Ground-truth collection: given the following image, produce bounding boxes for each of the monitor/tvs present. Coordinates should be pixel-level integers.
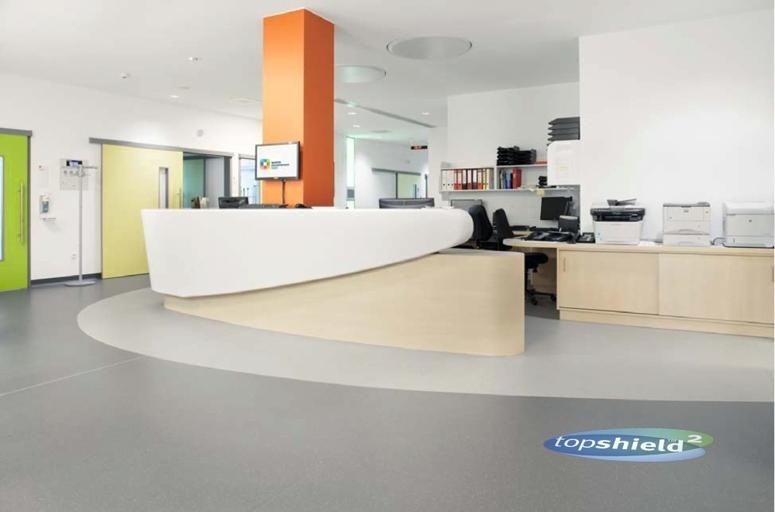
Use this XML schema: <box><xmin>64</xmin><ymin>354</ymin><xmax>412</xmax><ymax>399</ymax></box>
<box><xmin>219</xmin><ymin>197</ymin><xmax>249</xmax><ymax>208</ymax></box>
<box><xmin>379</xmin><ymin>197</ymin><xmax>435</xmax><ymax>209</ymax></box>
<box><xmin>540</xmin><ymin>196</ymin><xmax>570</xmax><ymax>220</ymax></box>
<box><xmin>451</xmin><ymin>199</ymin><xmax>482</xmax><ymax>212</ymax></box>
<box><xmin>255</xmin><ymin>142</ymin><xmax>300</xmax><ymax>180</ymax></box>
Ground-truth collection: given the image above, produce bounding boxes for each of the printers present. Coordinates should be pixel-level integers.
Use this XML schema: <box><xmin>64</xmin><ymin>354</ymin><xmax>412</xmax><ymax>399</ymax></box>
<box><xmin>590</xmin><ymin>207</ymin><xmax>645</xmax><ymax>244</ymax></box>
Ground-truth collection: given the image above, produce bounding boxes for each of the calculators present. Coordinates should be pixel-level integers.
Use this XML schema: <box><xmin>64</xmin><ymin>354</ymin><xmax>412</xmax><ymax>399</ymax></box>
<box><xmin>577</xmin><ymin>233</ymin><xmax>595</xmax><ymax>243</ymax></box>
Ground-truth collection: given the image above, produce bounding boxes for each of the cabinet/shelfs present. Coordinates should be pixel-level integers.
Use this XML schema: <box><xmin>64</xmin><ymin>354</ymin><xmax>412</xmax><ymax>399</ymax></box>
<box><xmin>656</xmin><ymin>254</ymin><xmax>772</xmax><ymax>341</ymax></box>
<box><xmin>439</xmin><ymin>160</ymin><xmax>576</xmax><ymax>192</ymax></box>
<box><xmin>557</xmin><ymin>251</ymin><xmax>657</xmax><ymax>329</ymax></box>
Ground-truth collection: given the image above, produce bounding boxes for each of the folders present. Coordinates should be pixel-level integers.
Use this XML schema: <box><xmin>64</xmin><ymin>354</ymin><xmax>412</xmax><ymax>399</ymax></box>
<box><xmin>441</xmin><ymin>169</ymin><xmax>494</xmax><ymax>190</ymax></box>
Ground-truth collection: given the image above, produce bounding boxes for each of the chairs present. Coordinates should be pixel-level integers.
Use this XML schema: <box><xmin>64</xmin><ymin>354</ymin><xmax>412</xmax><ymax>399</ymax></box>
<box><xmin>467</xmin><ymin>206</ymin><xmax>555</xmax><ymax>305</ymax></box>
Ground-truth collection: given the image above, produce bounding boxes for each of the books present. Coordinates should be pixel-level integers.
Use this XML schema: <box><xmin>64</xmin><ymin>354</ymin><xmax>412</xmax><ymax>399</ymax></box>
<box><xmin>442</xmin><ymin>168</ymin><xmax>522</xmax><ymax>190</ymax></box>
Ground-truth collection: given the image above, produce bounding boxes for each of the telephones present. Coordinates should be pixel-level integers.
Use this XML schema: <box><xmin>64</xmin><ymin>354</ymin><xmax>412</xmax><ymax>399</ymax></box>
<box><xmin>527</xmin><ymin>231</ymin><xmax>546</xmax><ymax>240</ymax></box>
